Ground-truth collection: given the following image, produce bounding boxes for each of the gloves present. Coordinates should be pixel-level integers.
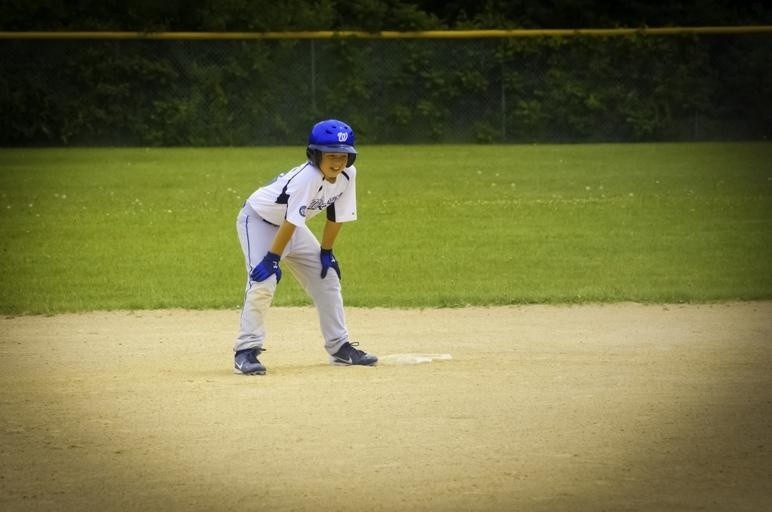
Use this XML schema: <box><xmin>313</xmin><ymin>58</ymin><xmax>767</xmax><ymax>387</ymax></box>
<box><xmin>250</xmin><ymin>252</ymin><xmax>282</xmax><ymax>283</ymax></box>
<box><xmin>319</xmin><ymin>246</ymin><xmax>342</xmax><ymax>280</ymax></box>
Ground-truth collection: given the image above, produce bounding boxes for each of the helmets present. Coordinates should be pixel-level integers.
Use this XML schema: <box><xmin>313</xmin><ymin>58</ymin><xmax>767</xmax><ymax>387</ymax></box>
<box><xmin>306</xmin><ymin>119</ymin><xmax>359</xmax><ymax>155</ymax></box>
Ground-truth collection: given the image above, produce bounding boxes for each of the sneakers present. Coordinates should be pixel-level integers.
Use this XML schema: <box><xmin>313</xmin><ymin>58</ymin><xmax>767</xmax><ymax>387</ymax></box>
<box><xmin>234</xmin><ymin>350</ymin><xmax>267</xmax><ymax>375</ymax></box>
<box><xmin>332</xmin><ymin>343</ymin><xmax>378</xmax><ymax>365</ymax></box>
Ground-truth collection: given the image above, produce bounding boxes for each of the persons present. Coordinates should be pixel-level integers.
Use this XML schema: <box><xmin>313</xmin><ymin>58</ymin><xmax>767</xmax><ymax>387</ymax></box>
<box><xmin>232</xmin><ymin>117</ymin><xmax>377</xmax><ymax>376</ymax></box>
